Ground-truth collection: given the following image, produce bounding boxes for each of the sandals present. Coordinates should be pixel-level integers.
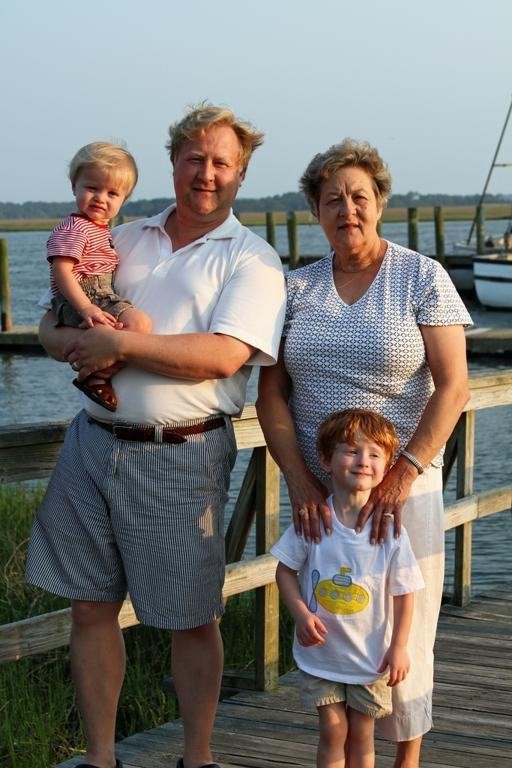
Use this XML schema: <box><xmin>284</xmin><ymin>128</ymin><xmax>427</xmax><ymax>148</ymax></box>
<box><xmin>72</xmin><ymin>372</ymin><xmax>118</xmax><ymax>413</ymax></box>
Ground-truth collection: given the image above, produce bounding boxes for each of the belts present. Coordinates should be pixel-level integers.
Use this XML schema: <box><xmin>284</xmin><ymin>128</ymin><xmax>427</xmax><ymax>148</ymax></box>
<box><xmin>81</xmin><ymin>408</ymin><xmax>231</xmax><ymax>444</ymax></box>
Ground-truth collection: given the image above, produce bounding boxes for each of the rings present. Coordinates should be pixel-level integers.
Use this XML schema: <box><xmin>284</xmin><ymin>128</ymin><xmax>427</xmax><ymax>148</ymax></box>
<box><xmin>383</xmin><ymin>513</ymin><xmax>393</xmax><ymax>517</ymax></box>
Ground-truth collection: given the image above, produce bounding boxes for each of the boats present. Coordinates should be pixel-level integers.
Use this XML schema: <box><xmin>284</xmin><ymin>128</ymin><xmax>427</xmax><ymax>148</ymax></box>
<box><xmin>423</xmin><ymin>228</ymin><xmax>511</xmax><ymax>308</ymax></box>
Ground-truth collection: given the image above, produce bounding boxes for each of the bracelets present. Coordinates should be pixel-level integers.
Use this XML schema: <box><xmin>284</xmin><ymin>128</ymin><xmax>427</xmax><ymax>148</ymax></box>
<box><xmin>400</xmin><ymin>451</ymin><xmax>423</xmax><ymax>475</ymax></box>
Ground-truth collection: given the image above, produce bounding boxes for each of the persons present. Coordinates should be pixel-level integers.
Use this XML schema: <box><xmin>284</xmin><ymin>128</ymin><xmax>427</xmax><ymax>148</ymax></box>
<box><xmin>46</xmin><ymin>141</ymin><xmax>152</xmax><ymax>413</ymax></box>
<box><xmin>254</xmin><ymin>138</ymin><xmax>473</xmax><ymax>768</ymax></box>
<box><xmin>268</xmin><ymin>409</ymin><xmax>425</xmax><ymax>766</ymax></box>
<box><xmin>36</xmin><ymin>99</ymin><xmax>287</xmax><ymax>767</ymax></box>
